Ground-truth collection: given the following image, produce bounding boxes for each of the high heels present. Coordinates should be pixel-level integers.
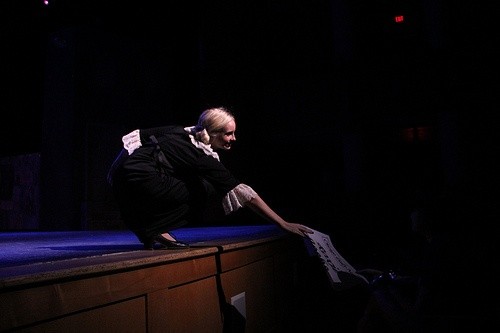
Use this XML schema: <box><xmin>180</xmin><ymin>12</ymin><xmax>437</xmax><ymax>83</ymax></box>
<box><xmin>143</xmin><ymin>234</ymin><xmax>189</xmax><ymax>249</ymax></box>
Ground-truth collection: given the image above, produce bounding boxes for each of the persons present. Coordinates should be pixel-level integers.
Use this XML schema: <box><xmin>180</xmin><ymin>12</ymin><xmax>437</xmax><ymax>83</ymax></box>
<box><xmin>107</xmin><ymin>104</ymin><xmax>313</xmax><ymax>251</ymax></box>
<box><xmin>357</xmin><ymin>189</ymin><xmax>470</xmax><ymax>333</ymax></box>
